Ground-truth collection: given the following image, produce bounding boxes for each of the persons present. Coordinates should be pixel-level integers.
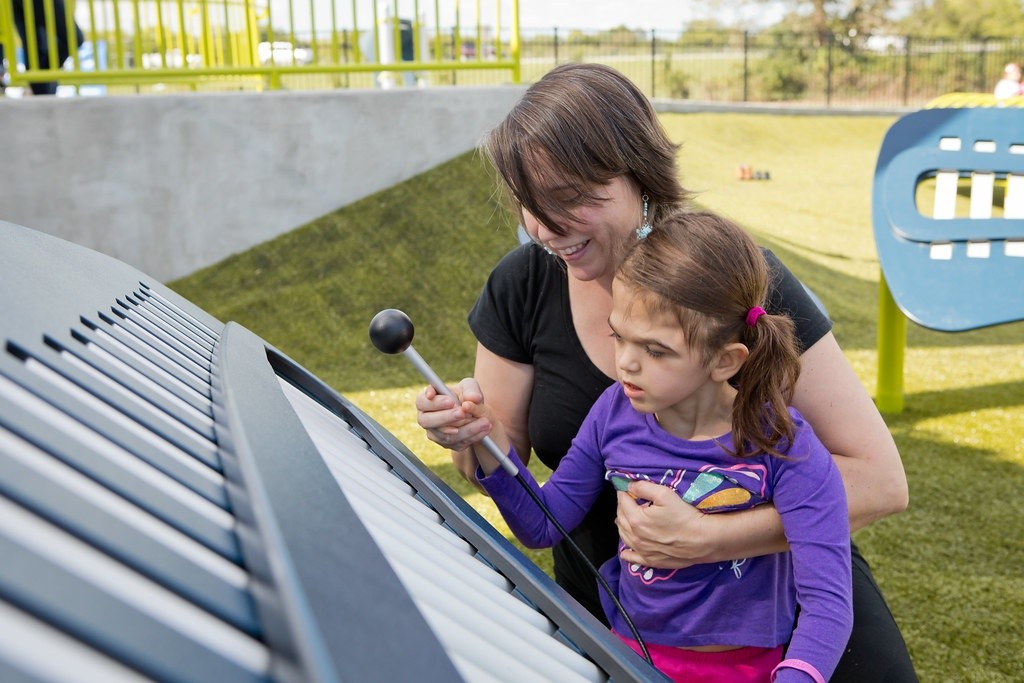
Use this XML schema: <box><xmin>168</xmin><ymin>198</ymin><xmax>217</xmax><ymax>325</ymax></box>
<box><xmin>993</xmin><ymin>62</ymin><xmax>1024</xmax><ymax>109</ymax></box>
<box><xmin>424</xmin><ymin>212</ymin><xmax>854</xmax><ymax>682</ymax></box>
<box><xmin>397</xmin><ymin>17</ymin><xmax>419</xmax><ymax>88</ymax></box>
<box><xmin>416</xmin><ymin>63</ymin><xmax>919</xmax><ymax>683</ymax></box>
<box><xmin>4</xmin><ymin>0</ymin><xmax>85</xmax><ymax>95</ymax></box>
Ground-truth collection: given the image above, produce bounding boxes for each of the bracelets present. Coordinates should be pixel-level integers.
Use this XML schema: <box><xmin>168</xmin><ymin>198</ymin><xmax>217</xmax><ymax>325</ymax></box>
<box><xmin>770</xmin><ymin>658</ymin><xmax>826</xmax><ymax>682</ymax></box>
